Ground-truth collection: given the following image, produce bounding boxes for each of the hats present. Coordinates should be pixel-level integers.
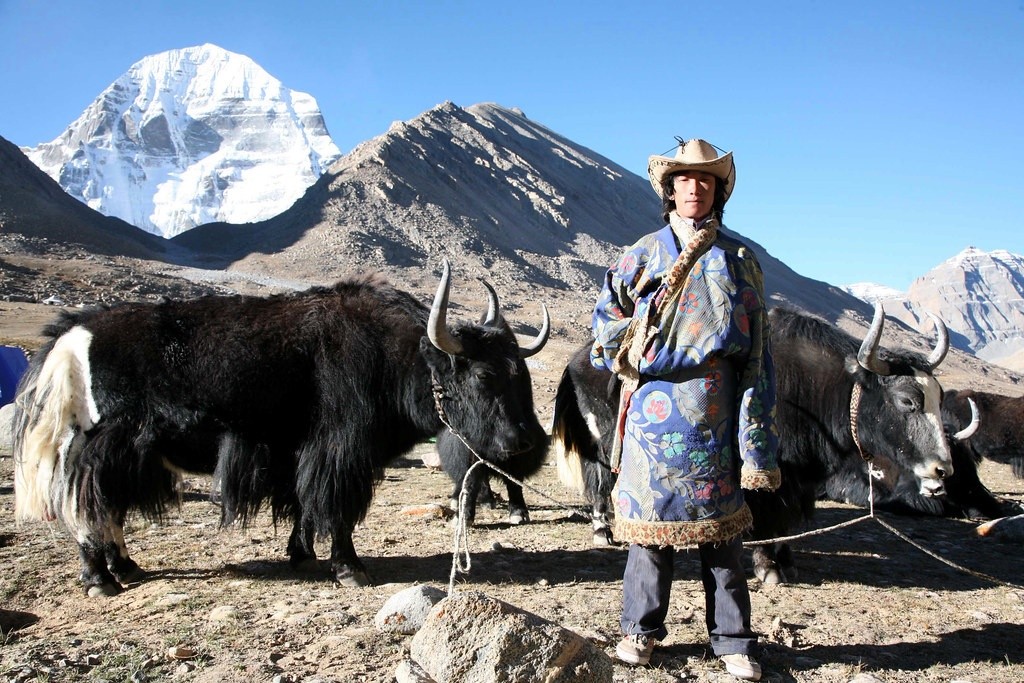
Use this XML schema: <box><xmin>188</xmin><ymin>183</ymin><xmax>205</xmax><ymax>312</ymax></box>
<box><xmin>647</xmin><ymin>135</ymin><xmax>736</xmax><ymax>204</ymax></box>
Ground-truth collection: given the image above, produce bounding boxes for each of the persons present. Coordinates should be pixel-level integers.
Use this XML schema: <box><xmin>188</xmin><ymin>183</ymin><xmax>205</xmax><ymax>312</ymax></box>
<box><xmin>589</xmin><ymin>139</ymin><xmax>783</xmax><ymax>683</ymax></box>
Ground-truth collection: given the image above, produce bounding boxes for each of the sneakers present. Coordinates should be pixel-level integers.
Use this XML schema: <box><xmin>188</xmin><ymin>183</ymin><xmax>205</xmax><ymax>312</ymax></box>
<box><xmin>718</xmin><ymin>654</ymin><xmax>762</xmax><ymax>680</ymax></box>
<box><xmin>615</xmin><ymin>632</ymin><xmax>654</xmax><ymax>666</ymax></box>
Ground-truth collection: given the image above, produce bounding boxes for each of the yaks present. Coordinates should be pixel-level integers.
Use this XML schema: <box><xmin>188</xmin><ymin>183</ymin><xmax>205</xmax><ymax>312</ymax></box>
<box><xmin>554</xmin><ymin>301</ymin><xmax>1024</xmax><ymax>594</ymax></box>
<box><xmin>12</xmin><ymin>259</ymin><xmax>550</xmax><ymax>601</ymax></box>
